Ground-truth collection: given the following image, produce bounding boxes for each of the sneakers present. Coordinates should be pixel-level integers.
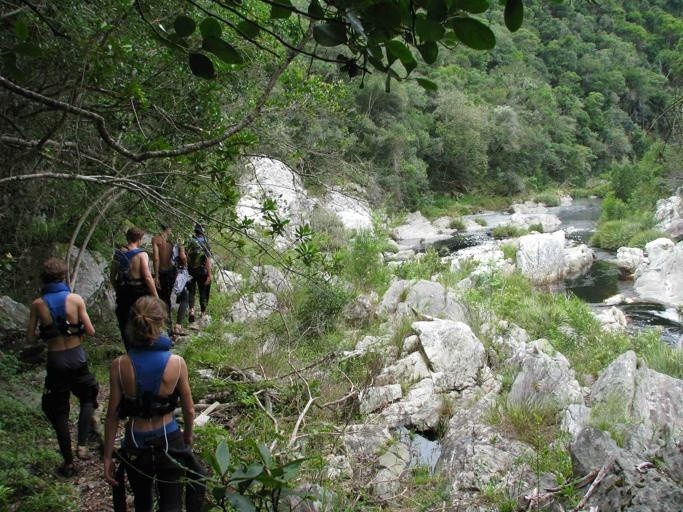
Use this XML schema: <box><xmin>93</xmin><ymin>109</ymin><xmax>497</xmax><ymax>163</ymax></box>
<box><xmin>174</xmin><ymin>327</ymin><xmax>187</xmax><ymax>336</ymax></box>
<box><xmin>170</xmin><ymin>336</ymin><xmax>181</xmax><ymax>346</ymax></box>
<box><xmin>76</xmin><ymin>445</ymin><xmax>93</xmax><ymax>459</ymax></box>
<box><xmin>58</xmin><ymin>462</ymin><xmax>75</xmax><ymax>476</ymax></box>
<box><xmin>190</xmin><ymin>312</ymin><xmax>195</xmax><ymax>323</ymax></box>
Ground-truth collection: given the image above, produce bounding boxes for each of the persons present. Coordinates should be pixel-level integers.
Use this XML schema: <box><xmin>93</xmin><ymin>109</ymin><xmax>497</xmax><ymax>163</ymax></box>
<box><xmin>103</xmin><ymin>295</ymin><xmax>195</xmax><ymax>511</ymax></box>
<box><xmin>26</xmin><ymin>256</ymin><xmax>98</xmax><ymax>477</ymax></box>
<box><xmin>184</xmin><ymin>224</ymin><xmax>212</xmax><ymax>322</ymax></box>
<box><xmin>151</xmin><ymin>219</ymin><xmax>189</xmax><ymax>346</ymax></box>
<box><xmin>108</xmin><ymin>227</ymin><xmax>157</xmax><ymax>353</ymax></box>
